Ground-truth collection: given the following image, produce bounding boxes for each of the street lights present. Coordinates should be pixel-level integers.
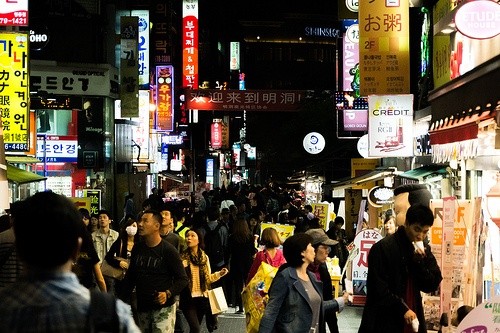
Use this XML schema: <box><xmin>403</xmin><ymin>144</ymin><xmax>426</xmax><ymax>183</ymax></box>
<box><xmin>184</xmin><ymin>86</ymin><xmax>207</xmax><ymax>227</ymax></box>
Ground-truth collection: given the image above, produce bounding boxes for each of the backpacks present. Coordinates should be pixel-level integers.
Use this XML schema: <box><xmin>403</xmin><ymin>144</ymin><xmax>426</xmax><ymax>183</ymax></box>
<box><xmin>204</xmin><ymin>223</ymin><xmax>223</xmax><ymax>262</ymax></box>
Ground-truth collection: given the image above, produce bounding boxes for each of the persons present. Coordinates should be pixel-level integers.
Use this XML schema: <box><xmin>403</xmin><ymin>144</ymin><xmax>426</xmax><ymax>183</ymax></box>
<box><xmin>302</xmin><ymin>228</ymin><xmax>340</xmax><ymax>333</ymax></box>
<box><xmin>358</xmin><ymin>204</ymin><xmax>443</xmax><ymax>333</ymax></box>
<box><xmin>148</xmin><ymin>182</ymin><xmax>315</xmax><ymax>317</ymax></box>
<box><xmin>0</xmin><ymin>190</ymin><xmax>141</xmax><ymax>333</ymax></box>
<box><xmin>258</xmin><ymin>233</ymin><xmax>355</xmax><ymax>333</ymax></box>
<box><xmin>1</xmin><ymin>193</ymin><xmax>230</xmax><ymax>333</ymax></box>
<box><xmin>325</xmin><ymin>215</ymin><xmax>350</xmax><ymax>271</ymax></box>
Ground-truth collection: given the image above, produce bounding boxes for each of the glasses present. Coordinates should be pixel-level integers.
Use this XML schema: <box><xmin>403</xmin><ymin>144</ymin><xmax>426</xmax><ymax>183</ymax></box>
<box><xmin>315</xmin><ymin>246</ymin><xmax>331</xmax><ymax>252</ymax></box>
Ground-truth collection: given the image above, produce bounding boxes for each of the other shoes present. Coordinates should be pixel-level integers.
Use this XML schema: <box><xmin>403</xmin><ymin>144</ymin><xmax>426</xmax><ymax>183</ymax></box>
<box><xmin>235</xmin><ymin>311</ymin><xmax>244</xmax><ymax>314</ymax></box>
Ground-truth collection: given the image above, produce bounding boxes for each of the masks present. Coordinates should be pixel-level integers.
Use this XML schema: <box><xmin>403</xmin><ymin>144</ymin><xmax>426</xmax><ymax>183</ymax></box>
<box><xmin>126</xmin><ymin>226</ymin><xmax>138</xmax><ymax>236</ymax></box>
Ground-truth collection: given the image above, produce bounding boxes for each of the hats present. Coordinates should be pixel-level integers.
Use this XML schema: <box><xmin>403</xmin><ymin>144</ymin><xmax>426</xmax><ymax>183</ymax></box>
<box><xmin>305</xmin><ymin>229</ymin><xmax>339</xmax><ymax>245</ymax></box>
<box><xmin>221</xmin><ymin>208</ymin><xmax>229</xmax><ymax>214</ymax></box>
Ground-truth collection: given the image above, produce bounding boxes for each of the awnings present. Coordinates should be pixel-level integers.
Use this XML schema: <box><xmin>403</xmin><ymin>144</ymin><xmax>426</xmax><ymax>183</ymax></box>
<box><xmin>7</xmin><ymin>164</ymin><xmax>47</xmax><ymax>187</ymax></box>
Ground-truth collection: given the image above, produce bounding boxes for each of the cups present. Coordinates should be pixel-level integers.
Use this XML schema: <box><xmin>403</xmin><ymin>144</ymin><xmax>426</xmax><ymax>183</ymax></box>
<box><xmin>412</xmin><ymin>240</ymin><xmax>424</xmax><ymax>254</ymax></box>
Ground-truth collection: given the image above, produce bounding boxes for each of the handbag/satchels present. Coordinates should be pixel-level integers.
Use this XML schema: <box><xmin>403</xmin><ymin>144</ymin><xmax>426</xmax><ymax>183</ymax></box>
<box><xmin>100</xmin><ymin>256</ymin><xmax>131</xmax><ymax>281</ymax></box>
<box><xmin>207</xmin><ymin>277</ymin><xmax>228</xmax><ymax>315</ymax></box>
